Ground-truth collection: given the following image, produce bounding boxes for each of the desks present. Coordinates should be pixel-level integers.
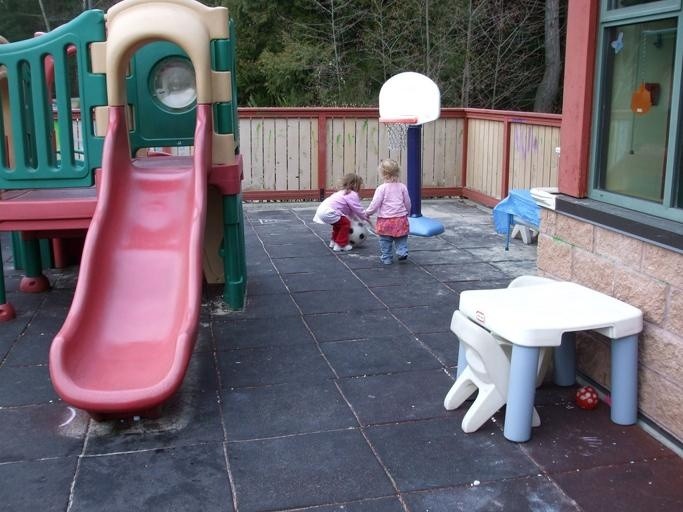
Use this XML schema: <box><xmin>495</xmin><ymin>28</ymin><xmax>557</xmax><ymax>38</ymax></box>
<box><xmin>460</xmin><ymin>282</ymin><xmax>642</xmax><ymax>444</ymax></box>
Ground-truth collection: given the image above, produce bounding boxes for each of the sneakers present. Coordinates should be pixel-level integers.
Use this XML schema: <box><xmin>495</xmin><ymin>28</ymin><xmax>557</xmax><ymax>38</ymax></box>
<box><xmin>329</xmin><ymin>240</ymin><xmax>353</xmax><ymax>252</ymax></box>
<box><xmin>398</xmin><ymin>254</ymin><xmax>407</xmax><ymax>261</ymax></box>
<box><xmin>380</xmin><ymin>256</ymin><xmax>393</xmax><ymax>265</ymax></box>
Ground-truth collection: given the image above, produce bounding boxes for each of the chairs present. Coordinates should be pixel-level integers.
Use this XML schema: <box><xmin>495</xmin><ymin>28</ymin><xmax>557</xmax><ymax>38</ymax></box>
<box><xmin>444</xmin><ymin>274</ymin><xmax>557</xmax><ymax>433</ymax></box>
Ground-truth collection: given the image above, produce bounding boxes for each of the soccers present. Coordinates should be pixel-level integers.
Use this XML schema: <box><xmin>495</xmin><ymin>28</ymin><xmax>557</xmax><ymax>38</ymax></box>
<box><xmin>349</xmin><ymin>220</ymin><xmax>367</xmax><ymax>246</ymax></box>
<box><xmin>575</xmin><ymin>386</ymin><xmax>598</xmax><ymax>409</ymax></box>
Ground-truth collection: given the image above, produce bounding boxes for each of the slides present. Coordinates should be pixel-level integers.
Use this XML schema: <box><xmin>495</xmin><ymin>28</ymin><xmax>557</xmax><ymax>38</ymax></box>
<box><xmin>47</xmin><ymin>107</ymin><xmax>212</xmax><ymax>414</ymax></box>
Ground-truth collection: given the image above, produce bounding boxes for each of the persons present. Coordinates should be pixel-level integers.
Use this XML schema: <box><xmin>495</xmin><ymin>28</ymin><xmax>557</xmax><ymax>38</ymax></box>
<box><xmin>312</xmin><ymin>172</ymin><xmax>373</xmax><ymax>252</ymax></box>
<box><xmin>365</xmin><ymin>158</ymin><xmax>412</xmax><ymax>265</ymax></box>
